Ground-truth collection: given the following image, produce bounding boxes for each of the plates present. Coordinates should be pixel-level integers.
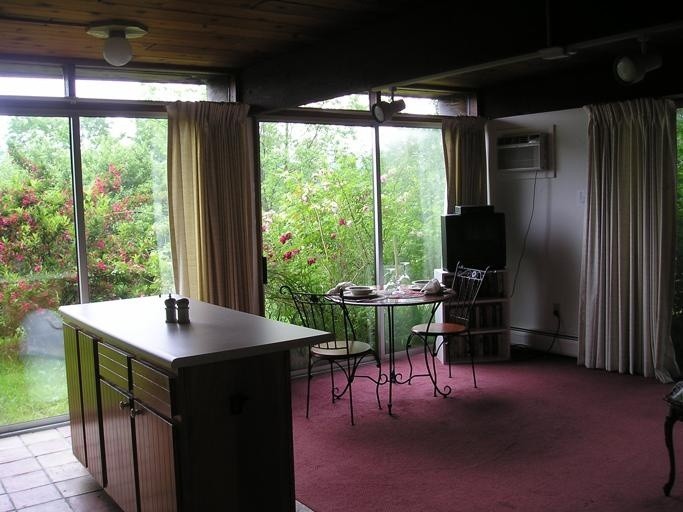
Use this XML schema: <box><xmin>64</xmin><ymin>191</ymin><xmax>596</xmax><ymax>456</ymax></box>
<box><xmin>343</xmin><ymin>290</ymin><xmax>377</xmax><ymax>298</ymax></box>
<box><xmin>411</xmin><ymin>284</ymin><xmax>446</xmax><ymax>291</ymax></box>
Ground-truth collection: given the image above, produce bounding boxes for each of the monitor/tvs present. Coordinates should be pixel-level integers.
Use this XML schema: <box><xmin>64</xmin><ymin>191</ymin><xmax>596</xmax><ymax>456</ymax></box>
<box><xmin>441</xmin><ymin>211</ymin><xmax>507</xmax><ymax>273</ymax></box>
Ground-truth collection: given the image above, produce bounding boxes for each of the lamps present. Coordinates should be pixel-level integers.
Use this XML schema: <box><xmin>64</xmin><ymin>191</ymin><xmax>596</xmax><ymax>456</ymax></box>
<box><xmin>371</xmin><ymin>86</ymin><xmax>405</xmax><ymax>123</ymax></box>
<box><xmin>617</xmin><ymin>54</ymin><xmax>640</xmax><ymax>83</ymax></box>
<box><xmin>81</xmin><ymin>21</ymin><xmax>149</xmax><ymax>67</ymax></box>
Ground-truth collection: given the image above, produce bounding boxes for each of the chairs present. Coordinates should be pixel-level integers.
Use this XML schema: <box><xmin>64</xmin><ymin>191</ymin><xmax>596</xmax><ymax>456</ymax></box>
<box><xmin>280</xmin><ymin>258</ymin><xmax>492</xmax><ymax>424</ymax></box>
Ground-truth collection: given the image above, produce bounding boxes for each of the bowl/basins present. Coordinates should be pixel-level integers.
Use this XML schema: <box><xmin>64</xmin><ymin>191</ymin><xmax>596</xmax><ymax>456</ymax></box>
<box><xmin>345</xmin><ymin>286</ymin><xmax>377</xmax><ymax>295</ymax></box>
<box><xmin>412</xmin><ymin>280</ymin><xmax>430</xmax><ymax>289</ymax></box>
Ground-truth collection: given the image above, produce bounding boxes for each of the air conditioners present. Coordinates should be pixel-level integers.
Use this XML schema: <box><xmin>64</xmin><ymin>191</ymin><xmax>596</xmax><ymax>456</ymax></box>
<box><xmin>490</xmin><ymin>132</ymin><xmax>546</xmax><ymax>172</ymax></box>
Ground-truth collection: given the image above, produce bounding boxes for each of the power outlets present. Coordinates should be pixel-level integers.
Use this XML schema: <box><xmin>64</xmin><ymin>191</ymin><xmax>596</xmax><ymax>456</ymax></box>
<box><xmin>553</xmin><ymin>300</ymin><xmax>563</xmax><ymax>317</ymax></box>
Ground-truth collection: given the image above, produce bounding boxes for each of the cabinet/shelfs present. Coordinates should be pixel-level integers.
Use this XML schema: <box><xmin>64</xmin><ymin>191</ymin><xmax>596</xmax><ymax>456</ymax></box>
<box><xmin>433</xmin><ymin>269</ymin><xmax>510</xmax><ymax>364</ymax></box>
<box><xmin>56</xmin><ymin>293</ymin><xmax>335</xmax><ymax>512</ymax></box>
